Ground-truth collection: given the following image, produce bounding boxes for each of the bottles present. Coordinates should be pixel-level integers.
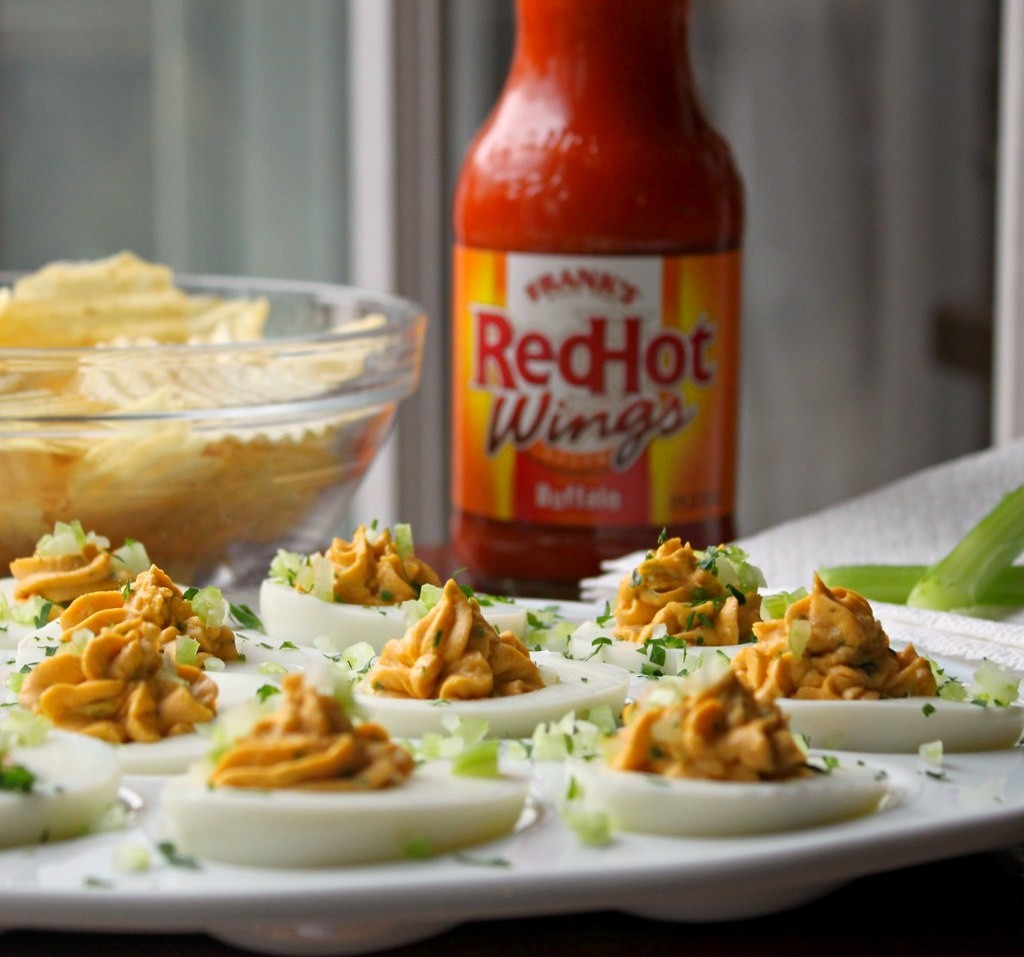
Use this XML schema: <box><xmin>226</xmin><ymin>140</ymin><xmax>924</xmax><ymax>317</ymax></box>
<box><xmin>445</xmin><ymin>0</ymin><xmax>750</xmax><ymax>600</ymax></box>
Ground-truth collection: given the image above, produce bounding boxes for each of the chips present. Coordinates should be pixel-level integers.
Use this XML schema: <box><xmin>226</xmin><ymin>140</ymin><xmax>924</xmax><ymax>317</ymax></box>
<box><xmin>2</xmin><ymin>249</ymin><xmax>390</xmax><ymax>563</ymax></box>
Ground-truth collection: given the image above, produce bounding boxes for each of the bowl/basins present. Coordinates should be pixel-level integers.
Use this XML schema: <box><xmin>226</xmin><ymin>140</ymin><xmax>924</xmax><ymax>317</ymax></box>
<box><xmin>0</xmin><ymin>269</ymin><xmax>428</xmax><ymax>597</ymax></box>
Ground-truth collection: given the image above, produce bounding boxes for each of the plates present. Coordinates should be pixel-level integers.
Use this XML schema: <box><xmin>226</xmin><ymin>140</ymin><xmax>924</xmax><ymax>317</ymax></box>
<box><xmin>0</xmin><ymin>592</ymin><xmax>1024</xmax><ymax>957</ymax></box>
